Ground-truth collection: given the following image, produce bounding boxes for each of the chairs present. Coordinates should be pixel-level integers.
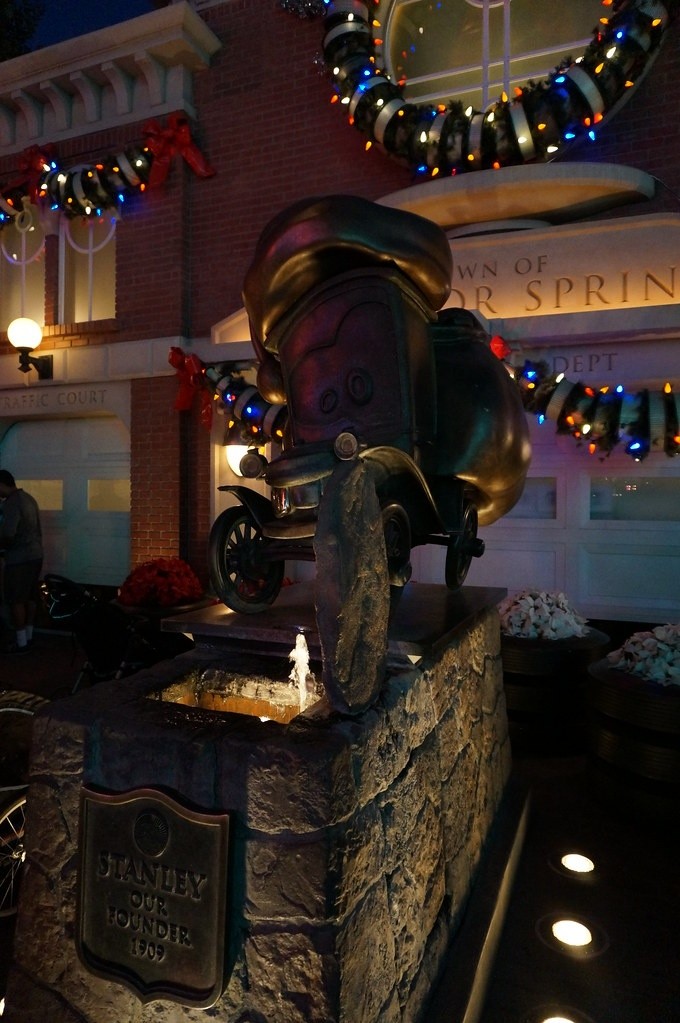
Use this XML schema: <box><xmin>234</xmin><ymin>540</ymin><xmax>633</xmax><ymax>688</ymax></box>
<box><xmin>43</xmin><ymin>573</ymin><xmax>156</xmax><ymax>694</ymax></box>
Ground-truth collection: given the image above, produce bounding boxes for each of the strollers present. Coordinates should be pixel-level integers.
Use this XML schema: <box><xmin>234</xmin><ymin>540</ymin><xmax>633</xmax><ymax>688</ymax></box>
<box><xmin>43</xmin><ymin>573</ymin><xmax>190</xmax><ymax>699</ymax></box>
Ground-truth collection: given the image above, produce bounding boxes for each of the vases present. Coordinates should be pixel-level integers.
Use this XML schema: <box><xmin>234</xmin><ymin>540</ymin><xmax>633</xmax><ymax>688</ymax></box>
<box><xmin>499</xmin><ymin>626</ymin><xmax>610</xmax><ymax>753</ymax></box>
<box><xmin>595</xmin><ymin>668</ymin><xmax>680</xmax><ymax>782</ymax></box>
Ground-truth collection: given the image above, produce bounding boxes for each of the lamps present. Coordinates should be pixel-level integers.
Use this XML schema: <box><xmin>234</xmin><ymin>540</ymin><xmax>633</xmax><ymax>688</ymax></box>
<box><xmin>547</xmin><ymin>848</ymin><xmax>601</xmax><ymax>900</ymax></box>
<box><xmin>519</xmin><ymin>1003</ymin><xmax>595</xmax><ymax>1023</ymax></box>
<box><xmin>535</xmin><ymin>910</ymin><xmax>610</xmax><ymax>967</ymax></box>
<box><xmin>7</xmin><ymin>318</ymin><xmax>53</xmax><ymax>380</ymax></box>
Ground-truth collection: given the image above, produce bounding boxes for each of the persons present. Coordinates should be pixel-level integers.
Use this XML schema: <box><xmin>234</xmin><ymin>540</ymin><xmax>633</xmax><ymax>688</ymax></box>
<box><xmin>0</xmin><ymin>470</ymin><xmax>43</xmax><ymax>657</ymax></box>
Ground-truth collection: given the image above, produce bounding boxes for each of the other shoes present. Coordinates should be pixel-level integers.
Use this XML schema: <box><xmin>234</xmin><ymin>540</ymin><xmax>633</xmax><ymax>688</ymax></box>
<box><xmin>2</xmin><ymin>644</ymin><xmax>32</xmax><ymax>656</ymax></box>
<box><xmin>7</xmin><ymin>640</ymin><xmax>35</xmax><ymax>646</ymax></box>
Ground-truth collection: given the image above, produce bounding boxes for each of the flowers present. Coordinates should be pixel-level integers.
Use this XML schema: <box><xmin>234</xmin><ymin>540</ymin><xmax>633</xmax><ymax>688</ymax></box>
<box><xmin>119</xmin><ymin>557</ymin><xmax>203</xmax><ymax>606</ymax></box>
<box><xmin>607</xmin><ymin>622</ymin><xmax>680</xmax><ymax>686</ymax></box>
<box><xmin>499</xmin><ymin>587</ymin><xmax>589</xmax><ymax>639</ymax></box>
